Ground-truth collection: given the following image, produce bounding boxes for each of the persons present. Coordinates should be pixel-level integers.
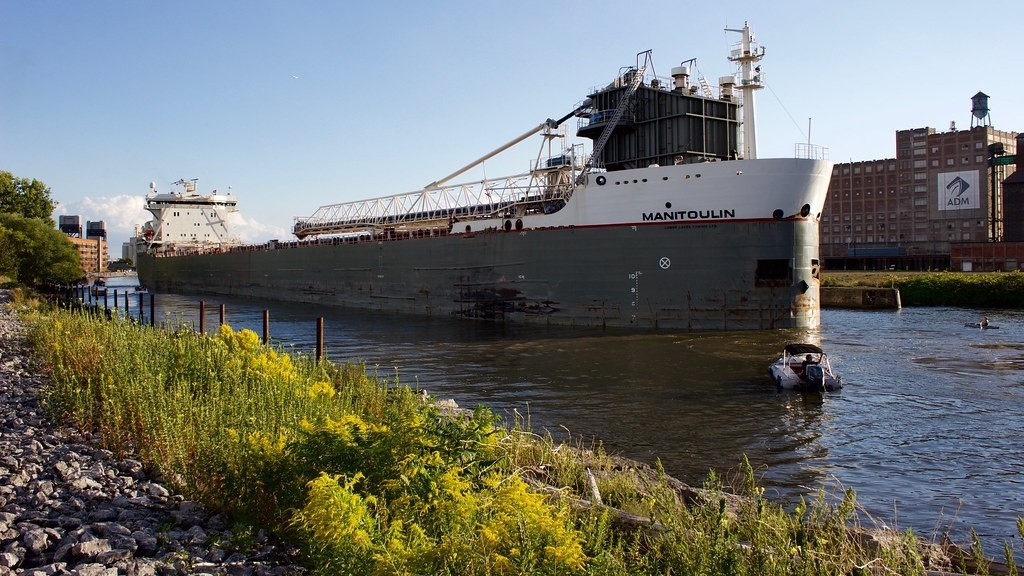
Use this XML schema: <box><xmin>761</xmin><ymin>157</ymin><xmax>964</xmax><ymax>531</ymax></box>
<box><xmin>799</xmin><ymin>353</ymin><xmax>815</xmax><ymax>379</ymax></box>
<box><xmin>135</xmin><ymin>284</ymin><xmax>146</xmax><ymax>291</ymax></box>
<box><xmin>977</xmin><ymin>316</ymin><xmax>990</xmax><ymax>326</ymax></box>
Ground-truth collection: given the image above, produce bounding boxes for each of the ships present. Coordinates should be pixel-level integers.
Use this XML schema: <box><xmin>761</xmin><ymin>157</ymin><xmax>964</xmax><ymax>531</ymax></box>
<box><xmin>125</xmin><ymin>15</ymin><xmax>839</xmax><ymax>329</ymax></box>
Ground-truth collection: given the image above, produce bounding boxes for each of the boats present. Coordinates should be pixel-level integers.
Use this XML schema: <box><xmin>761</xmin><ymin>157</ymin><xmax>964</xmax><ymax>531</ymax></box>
<box><xmin>767</xmin><ymin>343</ymin><xmax>842</xmax><ymax>394</ymax></box>
<box><xmin>91</xmin><ymin>275</ymin><xmax>108</xmax><ymax>296</ymax></box>
<box><xmin>965</xmin><ymin>322</ymin><xmax>1000</xmax><ymax>330</ymax></box>
